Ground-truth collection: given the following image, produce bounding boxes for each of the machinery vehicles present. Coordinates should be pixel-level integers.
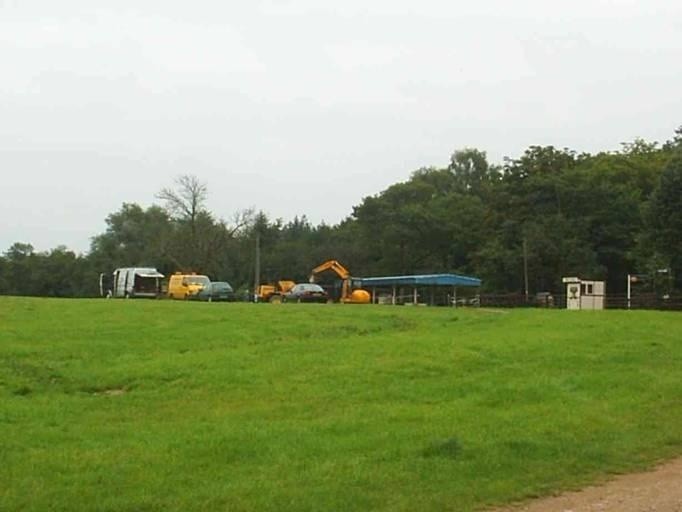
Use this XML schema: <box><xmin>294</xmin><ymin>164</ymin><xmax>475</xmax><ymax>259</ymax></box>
<box><xmin>308</xmin><ymin>259</ymin><xmax>371</xmax><ymax>304</ymax></box>
<box><xmin>257</xmin><ymin>280</ymin><xmax>297</xmax><ymax>305</ymax></box>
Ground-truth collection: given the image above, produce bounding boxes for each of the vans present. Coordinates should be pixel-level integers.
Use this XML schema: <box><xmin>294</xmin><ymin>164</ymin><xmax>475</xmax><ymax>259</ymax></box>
<box><xmin>99</xmin><ymin>267</ymin><xmax>166</xmax><ymax>298</ymax></box>
<box><xmin>167</xmin><ymin>271</ymin><xmax>211</xmax><ymax>300</ymax></box>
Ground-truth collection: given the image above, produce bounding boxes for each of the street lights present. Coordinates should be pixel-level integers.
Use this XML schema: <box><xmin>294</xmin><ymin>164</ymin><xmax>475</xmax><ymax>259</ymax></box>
<box><xmin>627</xmin><ymin>274</ymin><xmax>637</xmax><ymax>308</ymax></box>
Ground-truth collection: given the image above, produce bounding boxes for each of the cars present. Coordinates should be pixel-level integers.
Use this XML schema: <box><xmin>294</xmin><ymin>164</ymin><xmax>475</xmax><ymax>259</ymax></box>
<box><xmin>281</xmin><ymin>282</ymin><xmax>330</xmax><ymax>305</ymax></box>
<box><xmin>529</xmin><ymin>291</ymin><xmax>554</xmax><ymax>307</ymax></box>
<box><xmin>198</xmin><ymin>280</ymin><xmax>233</xmax><ymax>303</ymax></box>
